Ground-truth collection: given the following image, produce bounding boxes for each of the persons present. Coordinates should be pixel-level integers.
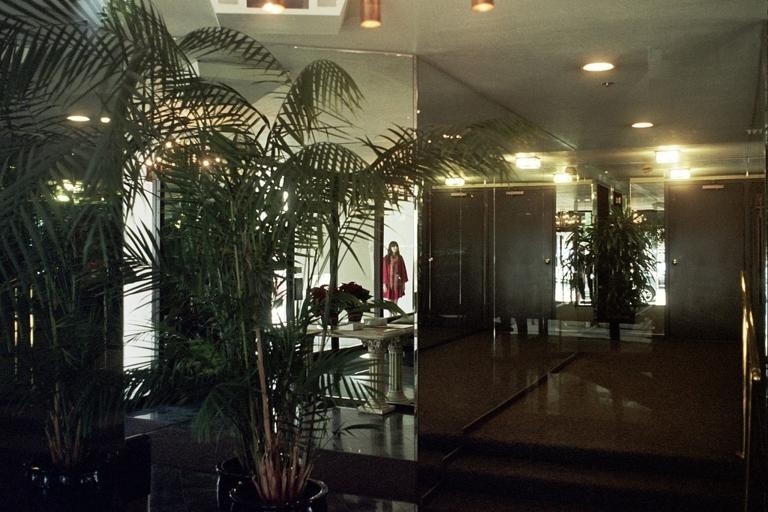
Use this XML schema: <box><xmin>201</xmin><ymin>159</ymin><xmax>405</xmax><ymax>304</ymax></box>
<box><xmin>382</xmin><ymin>240</ymin><xmax>407</xmax><ymax>314</ymax></box>
